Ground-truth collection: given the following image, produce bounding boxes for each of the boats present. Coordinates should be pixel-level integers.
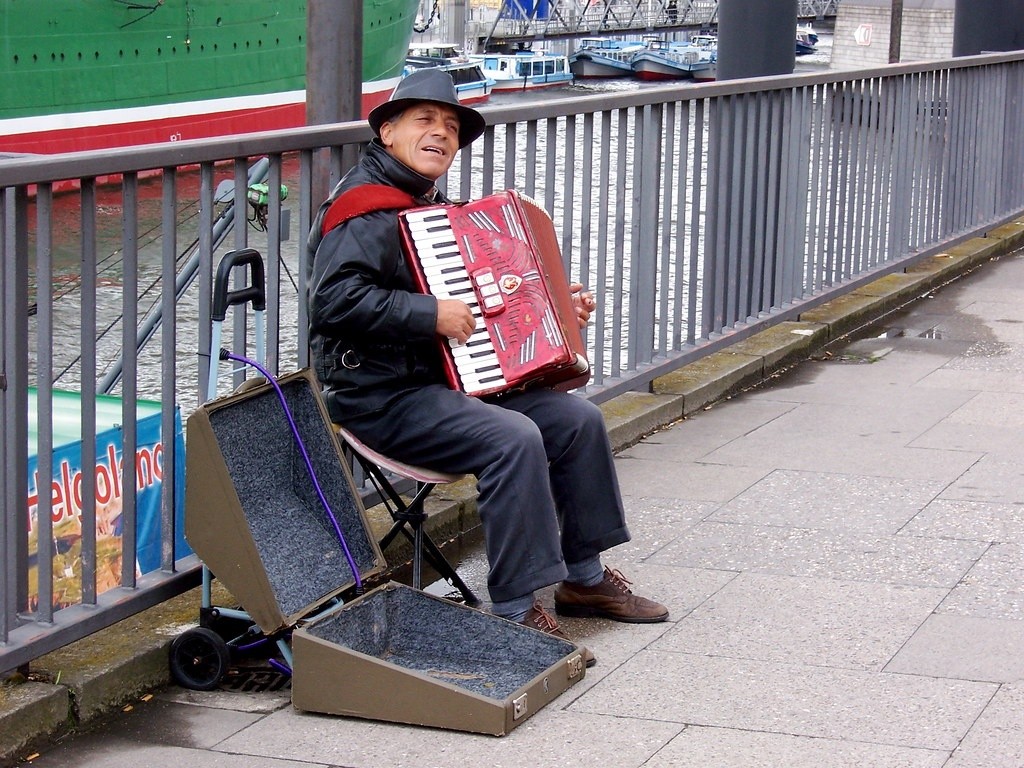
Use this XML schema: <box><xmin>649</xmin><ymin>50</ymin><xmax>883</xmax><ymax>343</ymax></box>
<box><xmin>399</xmin><ymin>0</ymin><xmax>820</xmax><ymax>106</ymax></box>
<box><xmin>0</xmin><ymin>0</ymin><xmax>423</xmax><ymax>202</ymax></box>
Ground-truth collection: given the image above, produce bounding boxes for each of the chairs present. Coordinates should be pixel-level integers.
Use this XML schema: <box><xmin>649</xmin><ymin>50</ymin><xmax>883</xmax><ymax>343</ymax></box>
<box><xmin>336</xmin><ymin>428</ymin><xmax>477</xmax><ymax>603</ymax></box>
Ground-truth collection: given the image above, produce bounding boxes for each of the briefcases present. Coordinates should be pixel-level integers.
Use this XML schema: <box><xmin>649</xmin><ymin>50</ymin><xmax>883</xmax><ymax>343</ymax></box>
<box><xmin>186</xmin><ymin>366</ymin><xmax>587</xmax><ymax>739</ymax></box>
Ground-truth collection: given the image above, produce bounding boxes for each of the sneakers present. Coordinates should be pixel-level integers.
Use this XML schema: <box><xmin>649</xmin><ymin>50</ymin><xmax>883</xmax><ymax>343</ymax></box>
<box><xmin>554</xmin><ymin>565</ymin><xmax>669</xmax><ymax>624</ymax></box>
<box><xmin>518</xmin><ymin>598</ymin><xmax>597</xmax><ymax>668</ymax></box>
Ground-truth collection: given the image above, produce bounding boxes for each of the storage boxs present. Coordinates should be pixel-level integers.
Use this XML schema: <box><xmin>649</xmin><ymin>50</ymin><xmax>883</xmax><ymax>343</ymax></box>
<box><xmin>183</xmin><ymin>367</ymin><xmax>586</xmax><ymax>737</ymax></box>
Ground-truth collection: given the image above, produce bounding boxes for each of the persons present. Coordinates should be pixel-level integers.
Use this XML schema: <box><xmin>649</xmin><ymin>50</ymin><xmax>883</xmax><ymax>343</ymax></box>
<box><xmin>304</xmin><ymin>68</ymin><xmax>669</xmax><ymax>666</ymax></box>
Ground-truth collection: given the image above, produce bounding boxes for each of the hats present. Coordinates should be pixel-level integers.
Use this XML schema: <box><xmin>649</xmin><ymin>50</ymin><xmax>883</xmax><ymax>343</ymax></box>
<box><xmin>367</xmin><ymin>69</ymin><xmax>486</xmax><ymax>150</ymax></box>
